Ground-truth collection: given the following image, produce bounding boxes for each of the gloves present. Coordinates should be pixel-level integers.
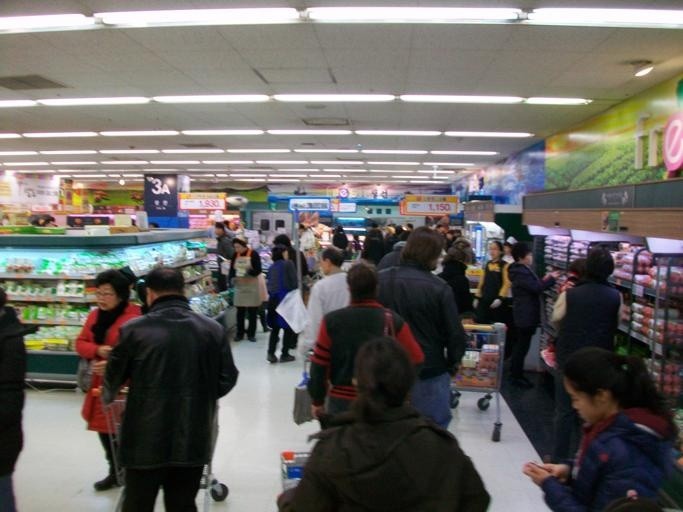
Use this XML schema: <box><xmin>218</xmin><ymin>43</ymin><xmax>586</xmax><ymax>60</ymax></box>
<box><xmin>490</xmin><ymin>299</ymin><xmax>502</xmax><ymax>309</ymax></box>
<box><xmin>472</xmin><ymin>299</ymin><xmax>480</xmax><ymax>309</ymax></box>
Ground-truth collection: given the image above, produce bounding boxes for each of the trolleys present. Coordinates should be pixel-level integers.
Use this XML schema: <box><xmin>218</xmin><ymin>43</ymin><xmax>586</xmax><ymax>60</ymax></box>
<box><xmin>279</xmin><ymin>450</ymin><xmax>313</xmax><ymax>492</ymax></box>
<box><xmin>93</xmin><ymin>386</ymin><xmax>228</xmax><ymax>511</ymax></box>
<box><xmin>442</xmin><ymin>322</ymin><xmax>506</xmax><ymax>443</ymax></box>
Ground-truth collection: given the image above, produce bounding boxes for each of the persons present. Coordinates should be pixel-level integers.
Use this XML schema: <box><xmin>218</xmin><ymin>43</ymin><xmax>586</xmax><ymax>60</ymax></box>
<box><xmin>522</xmin><ymin>346</ymin><xmax>680</xmax><ymax>511</ymax></box>
<box><xmin>28</xmin><ymin>214</ymin><xmax>240</xmax><ymax>511</ymax></box>
<box><xmin>601</xmin><ymin>494</ymin><xmax>662</xmax><ymax>511</ymax></box>
<box><xmin>215</xmin><ymin>214</ymin><xmax>623</xmax><ymax>511</ymax></box>
<box><xmin>0</xmin><ymin>284</ymin><xmax>41</xmax><ymax>511</ymax></box>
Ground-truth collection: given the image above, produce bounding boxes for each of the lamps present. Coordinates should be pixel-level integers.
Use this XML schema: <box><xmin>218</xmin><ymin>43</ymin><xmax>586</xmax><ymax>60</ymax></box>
<box><xmin>0</xmin><ymin>129</ymin><xmax>534</xmax><ymax>186</ymax></box>
<box><xmin>0</xmin><ymin>6</ymin><xmax>682</xmax><ymax>37</ymax></box>
<box><xmin>631</xmin><ymin>62</ymin><xmax>653</xmax><ymax>78</ymax></box>
<box><xmin>0</xmin><ymin>94</ymin><xmax>592</xmax><ymax>111</ymax></box>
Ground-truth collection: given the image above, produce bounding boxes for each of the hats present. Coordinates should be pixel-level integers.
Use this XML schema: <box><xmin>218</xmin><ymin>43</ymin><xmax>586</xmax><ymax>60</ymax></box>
<box><xmin>507</xmin><ymin>236</ymin><xmax>517</xmax><ymax>245</ymax></box>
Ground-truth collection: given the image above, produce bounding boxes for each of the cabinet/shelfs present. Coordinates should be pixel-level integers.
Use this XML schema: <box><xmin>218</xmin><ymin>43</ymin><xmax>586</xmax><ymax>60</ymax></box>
<box><xmin>0</xmin><ymin>230</ymin><xmax>233</xmax><ymax>387</ymax></box>
<box><xmin>539</xmin><ymin>237</ymin><xmax>682</xmax><ymax>404</ymax></box>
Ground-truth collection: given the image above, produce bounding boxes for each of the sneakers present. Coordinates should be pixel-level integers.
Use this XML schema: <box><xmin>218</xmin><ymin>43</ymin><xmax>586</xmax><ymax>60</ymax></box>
<box><xmin>267</xmin><ymin>353</ymin><xmax>277</xmax><ymax>362</ymax></box>
<box><xmin>540</xmin><ymin>349</ymin><xmax>556</xmax><ymax>367</ymax></box>
<box><xmin>280</xmin><ymin>354</ymin><xmax>295</xmax><ymax>362</ymax></box>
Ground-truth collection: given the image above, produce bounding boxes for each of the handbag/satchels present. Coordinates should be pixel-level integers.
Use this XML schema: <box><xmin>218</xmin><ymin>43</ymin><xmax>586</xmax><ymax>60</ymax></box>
<box><xmin>77</xmin><ymin>358</ymin><xmax>92</xmax><ymax>393</ymax></box>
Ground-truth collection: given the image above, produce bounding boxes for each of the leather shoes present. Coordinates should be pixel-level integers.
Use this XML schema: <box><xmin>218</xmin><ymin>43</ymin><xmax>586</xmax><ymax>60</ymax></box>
<box><xmin>95</xmin><ymin>474</ymin><xmax>113</xmax><ymax>490</ymax></box>
